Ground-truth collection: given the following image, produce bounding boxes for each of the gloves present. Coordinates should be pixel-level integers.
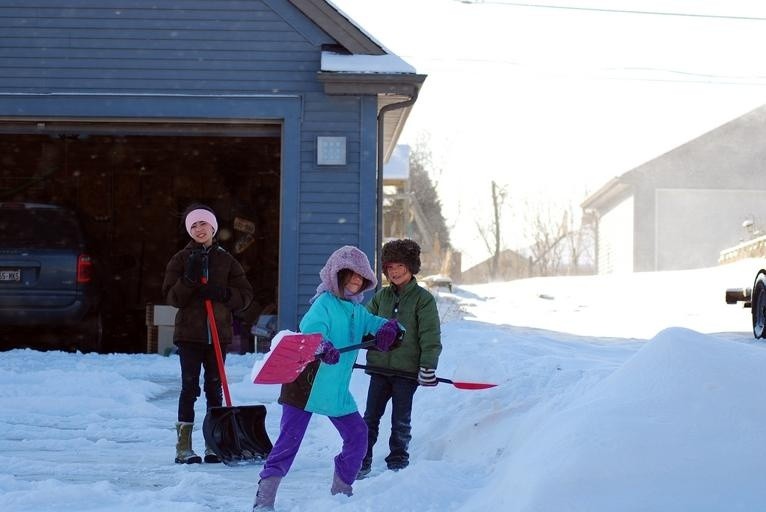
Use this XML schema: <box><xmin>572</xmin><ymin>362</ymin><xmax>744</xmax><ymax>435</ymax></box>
<box><xmin>318</xmin><ymin>339</ymin><xmax>340</xmax><ymax>365</ymax></box>
<box><xmin>374</xmin><ymin>319</ymin><xmax>400</xmax><ymax>352</ymax></box>
<box><xmin>417</xmin><ymin>369</ymin><xmax>438</xmax><ymax>387</ymax></box>
<box><xmin>183</xmin><ymin>253</ymin><xmax>203</xmax><ymax>283</ymax></box>
<box><xmin>197</xmin><ymin>282</ymin><xmax>229</xmax><ymax>302</ymax></box>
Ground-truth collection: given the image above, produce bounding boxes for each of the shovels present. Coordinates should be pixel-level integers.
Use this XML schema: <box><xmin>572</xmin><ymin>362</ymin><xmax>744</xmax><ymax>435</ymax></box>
<box><xmin>200</xmin><ymin>275</ymin><xmax>274</xmax><ymax>468</ymax></box>
<box><xmin>253</xmin><ymin>332</ymin><xmax>378</xmax><ymax>384</ymax></box>
<box><xmin>353</xmin><ymin>363</ymin><xmax>498</xmax><ymax>389</ymax></box>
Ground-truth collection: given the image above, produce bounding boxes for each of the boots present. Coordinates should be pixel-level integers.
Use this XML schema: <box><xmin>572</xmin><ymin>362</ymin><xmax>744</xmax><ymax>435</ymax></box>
<box><xmin>175</xmin><ymin>421</ymin><xmax>220</xmax><ymax>463</ymax></box>
<box><xmin>330</xmin><ymin>467</ymin><xmax>351</xmax><ymax>496</ymax></box>
<box><xmin>253</xmin><ymin>475</ymin><xmax>281</xmax><ymax>511</ymax></box>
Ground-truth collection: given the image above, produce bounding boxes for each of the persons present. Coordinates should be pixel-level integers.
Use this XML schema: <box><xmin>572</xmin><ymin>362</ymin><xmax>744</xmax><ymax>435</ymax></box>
<box><xmin>163</xmin><ymin>210</ymin><xmax>255</xmax><ymax>464</ymax></box>
<box><xmin>356</xmin><ymin>241</ymin><xmax>442</xmax><ymax>481</ymax></box>
<box><xmin>253</xmin><ymin>246</ymin><xmax>407</xmax><ymax>512</ymax></box>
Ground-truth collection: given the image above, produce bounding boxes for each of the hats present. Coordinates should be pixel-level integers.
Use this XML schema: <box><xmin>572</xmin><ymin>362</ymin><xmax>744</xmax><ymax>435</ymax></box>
<box><xmin>382</xmin><ymin>240</ymin><xmax>421</xmax><ymax>274</ymax></box>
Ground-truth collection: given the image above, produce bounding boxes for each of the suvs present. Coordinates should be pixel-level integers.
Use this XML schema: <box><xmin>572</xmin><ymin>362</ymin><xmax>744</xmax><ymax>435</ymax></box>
<box><xmin>0</xmin><ymin>200</ymin><xmax>113</xmax><ymax>327</ymax></box>
